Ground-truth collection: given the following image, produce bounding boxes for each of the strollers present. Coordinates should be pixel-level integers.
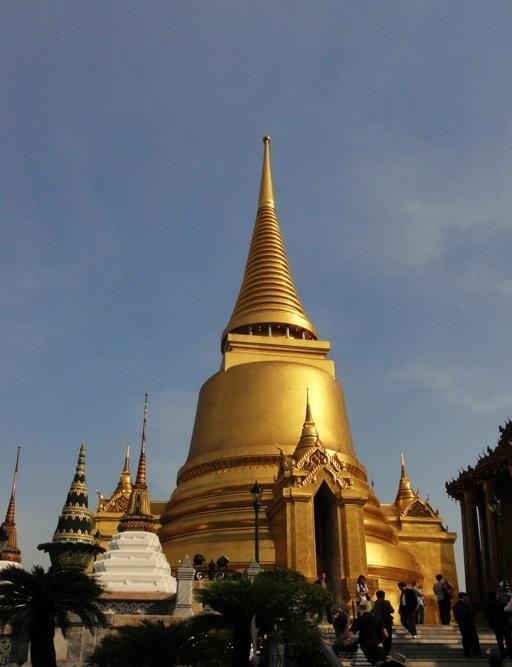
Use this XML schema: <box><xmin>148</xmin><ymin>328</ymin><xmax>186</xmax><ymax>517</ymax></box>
<box><xmin>333</xmin><ymin>609</ymin><xmax>359</xmax><ymax>658</ymax></box>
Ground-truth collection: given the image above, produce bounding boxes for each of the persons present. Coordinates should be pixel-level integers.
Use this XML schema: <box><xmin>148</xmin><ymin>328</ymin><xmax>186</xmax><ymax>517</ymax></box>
<box><xmin>346</xmin><ymin>598</ymin><xmax>389</xmax><ymax>666</ymax></box>
<box><xmin>328</xmin><ymin>605</ymin><xmax>359</xmax><ymax>660</ymax></box>
<box><xmin>374</xmin><ymin>588</ymin><xmax>394</xmax><ymax>637</ymax></box>
<box><xmin>432</xmin><ymin>573</ymin><xmax>455</xmax><ymax>625</ymax></box>
<box><xmin>410</xmin><ymin>579</ymin><xmax>427</xmax><ymax>624</ymax></box>
<box><xmin>397</xmin><ymin>581</ymin><xmax>419</xmax><ymax>638</ymax></box>
<box><xmin>453</xmin><ymin>575</ymin><xmax>511</xmax><ymax>667</ymax></box>
<box><xmin>355</xmin><ymin>575</ymin><xmax>372</xmax><ymax>617</ymax></box>
<box><xmin>313</xmin><ymin>570</ymin><xmax>334</xmax><ymax>625</ymax></box>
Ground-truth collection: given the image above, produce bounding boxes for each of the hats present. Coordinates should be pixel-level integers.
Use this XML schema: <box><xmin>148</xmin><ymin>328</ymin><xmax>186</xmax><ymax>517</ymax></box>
<box><xmin>355</xmin><ymin>600</ymin><xmax>375</xmax><ymax>612</ymax></box>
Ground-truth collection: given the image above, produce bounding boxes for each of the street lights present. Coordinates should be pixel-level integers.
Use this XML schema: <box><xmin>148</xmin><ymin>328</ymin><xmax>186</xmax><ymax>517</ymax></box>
<box><xmin>250</xmin><ymin>478</ymin><xmax>263</xmax><ymax>563</ymax></box>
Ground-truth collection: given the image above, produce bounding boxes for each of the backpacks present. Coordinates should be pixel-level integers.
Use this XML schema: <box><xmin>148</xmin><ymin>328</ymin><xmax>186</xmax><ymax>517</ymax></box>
<box><xmin>442</xmin><ymin>580</ymin><xmax>456</xmax><ymax>599</ymax></box>
